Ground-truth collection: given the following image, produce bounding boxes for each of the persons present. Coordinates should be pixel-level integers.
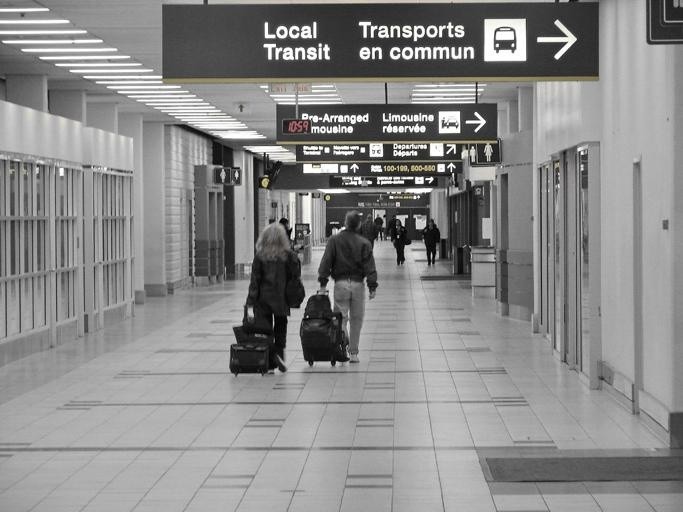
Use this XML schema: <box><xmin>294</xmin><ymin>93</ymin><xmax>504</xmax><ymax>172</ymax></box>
<box><xmin>381</xmin><ymin>215</ymin><xmax>389</xmax><ymax>241</ymax></box>
<box><xmin>387</xmin><ymin>215</ymin><xmax>395</xmax><ymax>241</ymax></box>
<box><xmin>315</xmin><ymin>210</ymin><xmax>377</xmax><ymax>362</ymax></box>
<box><xmin>420</xmin><ymin>219</ymin><xmax>441</xmax><ymax>266</ymax></box>
<box><xmin>279</xmin><ymin>217</ymin><xmax>293</xmax><ymax>246</ymax></box>
<box><xmin>243</xmin><ymin>222</ymin><xmax>302</xmax><ymax>372</ymax></box>
<box><xmin>358</xmin><ymin>213</ymin><xmax>378</xmax><ymax>251</ymax></box>
<box><xmin>389</xmin><ymin>218</ymin><xmax>407</xmax><ymax>266</ymax></box>
<box><xmin>374</xmin><ymin>214</ymin><xmax>383</xmax><ymax>241</ymax></box>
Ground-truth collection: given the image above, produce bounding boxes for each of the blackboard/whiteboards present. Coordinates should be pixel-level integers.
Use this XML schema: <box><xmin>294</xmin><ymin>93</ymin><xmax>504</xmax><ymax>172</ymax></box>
<box><xmin>295</xmin><ymin>224</ymin><xmax>310</xmax><ymax>250</ymax></box>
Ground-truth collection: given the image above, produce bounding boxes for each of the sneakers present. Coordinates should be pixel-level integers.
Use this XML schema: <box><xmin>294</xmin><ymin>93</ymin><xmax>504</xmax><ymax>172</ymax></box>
<box><xmin>273</xmin><ymin>344</ymin><xmax>288</xmax><ymax>372</ymax></box>
<box><xmin>348</xmin><ymin>352</ymin><xmax>361</xmax><ymax>363</ymax></box>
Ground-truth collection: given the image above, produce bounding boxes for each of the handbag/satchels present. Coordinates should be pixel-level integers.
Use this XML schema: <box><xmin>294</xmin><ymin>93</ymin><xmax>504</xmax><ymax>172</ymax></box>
<box><xmin>287</xmin><ymin>277</ymin><xmax>305</xmax><ymax>309</ymax></box>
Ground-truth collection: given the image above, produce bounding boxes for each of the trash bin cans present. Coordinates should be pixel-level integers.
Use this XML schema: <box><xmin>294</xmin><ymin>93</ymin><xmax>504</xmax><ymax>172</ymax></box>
<box><xmin>471</xmin><ymin>245</ymin><xmax>497</xmax><ymax>288</ymax></box>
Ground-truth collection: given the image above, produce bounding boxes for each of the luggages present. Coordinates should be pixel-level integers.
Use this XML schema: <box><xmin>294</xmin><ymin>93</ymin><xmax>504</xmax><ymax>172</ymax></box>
<box><xmin>300</xmin><ymin>295</ymin><xmax>342</xmax><ymax>365</ymax></box>
<box><xmin>229</xmin><ymin>303</ymin><xmax>272</xmax><ymax>374</ymax></box>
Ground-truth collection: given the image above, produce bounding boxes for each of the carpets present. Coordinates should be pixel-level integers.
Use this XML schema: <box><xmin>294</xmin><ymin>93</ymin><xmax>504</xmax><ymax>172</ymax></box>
<box><xmin>420</xmin><ymin>275</ymin><xmax>471</xmax><ymax>281</ymax></box>
<box><xmin>415</xmin><ymin>260</ymin><xmax>451</xmax><ymax>262</ymax></box>
<box><xmin>412</xmin><ymin>249</ymin><xmax>439</xmax><ymax>251</ymax></box>
<box><xmin>486</xmin><ymin>455</ymin><xmax>683</xmax><ymax>482</ymax></box>
